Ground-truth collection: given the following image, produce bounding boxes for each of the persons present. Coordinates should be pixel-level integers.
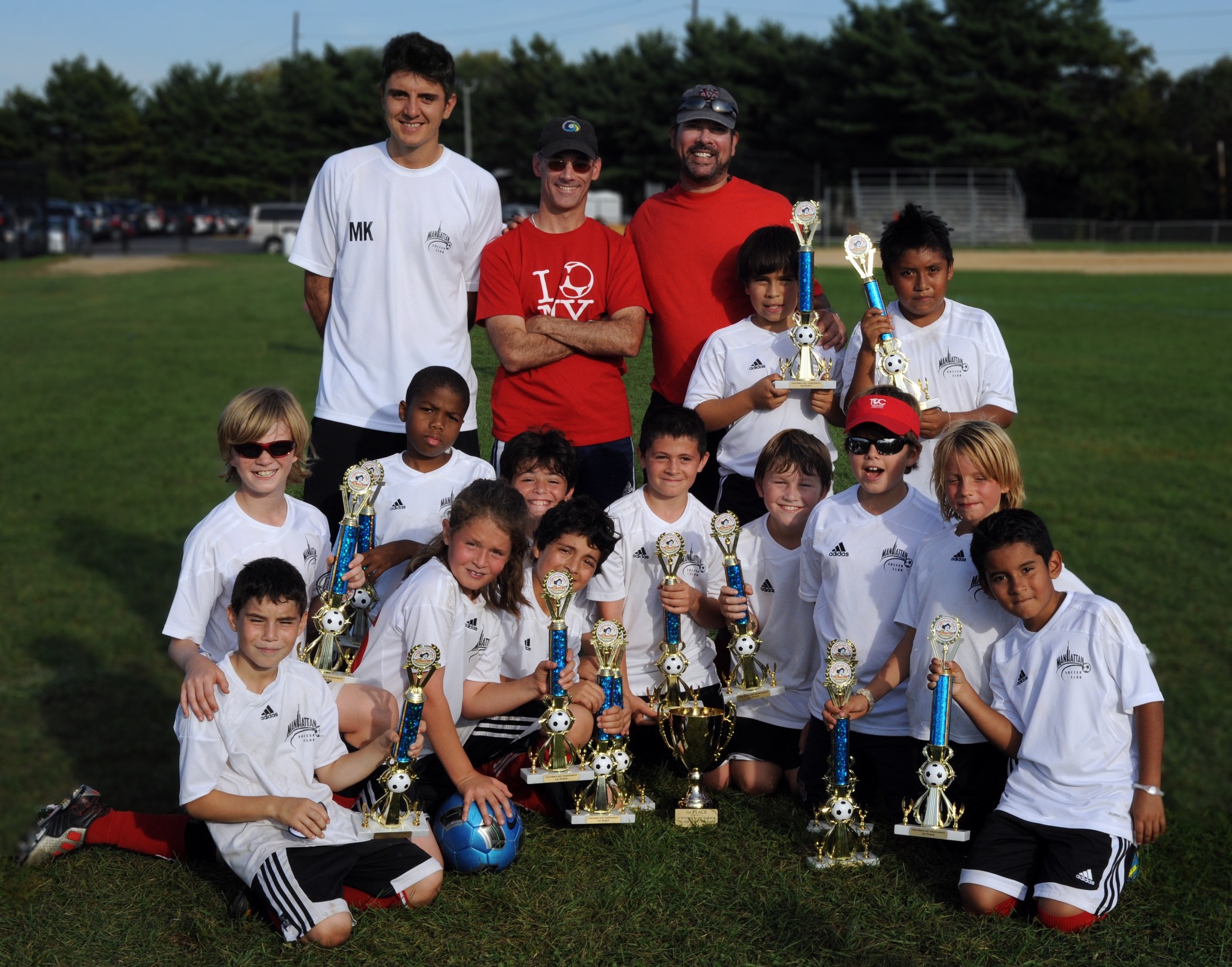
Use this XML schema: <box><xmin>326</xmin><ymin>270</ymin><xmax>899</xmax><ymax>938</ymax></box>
<box><xmin>488</xmin><ymin>79</ymin><xmax>847</xmax><ymax>525</ymax></box>
<box><xmin>839</xmin><ymin>199</ymin><xmax>1020</xmax><ymax>505</ymax></box>
<box><xmin>289</xmin><ymin>27</ymin><xmax>506</xmax><ymax>540</ymax></box>
<box><xmin>685</xmin><ymin>224</ymin><xmax>847</xmax><ymax>520</ymax></box>
<box><xmin>478</xmin><ymin>108</ymin><xmax>653</xmax><ymax>533</ymax></box>
<box><xmin>25</xmin><ymin>369</ymin><xmax>1179</xmax><ymax>959</ymax></box>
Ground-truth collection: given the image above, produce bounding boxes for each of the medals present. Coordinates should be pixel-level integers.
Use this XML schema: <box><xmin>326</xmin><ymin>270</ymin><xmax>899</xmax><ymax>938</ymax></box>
<box><xmin>808</xmin><ymin>638</ymin><xmax>872</xmax><ymax>837</ymax></box>
<box><xmin>349</xmin><ymin>644</ymin><xmax>444</xmax><ymax>838</ymax></box>
<box><xmin>642</xmin><ymin>532</ymin><xmax>707</xmax><ymax>722</ymax></box>
<box><xmin>807</xmin><ymin>658</ymin><xmax>881</xmax><ymax>872</ymax></box>
<box><xmin>895</xmin><ymin>615</ymin><xmax>973</xmax><ymax>841</ymax></box>
<box><xmin>519</xmin><ymin>568</ymin><xmax>596</xmax><ymax>785</ymax></box>
<box><xmin>565</xmin><ymin>618</ymin><xmax>636</xmax><ymax>824</ymax></box>
<box><xmin>710</xmin><ymin>511</ymin><xmax>785</xmax><ymax>705</ymax></box>
<box><xmin>336</xmin><ymin>458</ymin><xmax>384</xmax><ymax>651</ymax></box>
<box><xmin>297</xmin><ymin>465</ymin><xmax>373</xmax><ymax>683</ymax></box>
<box><xmin>657</xmin><ymin>698</ymin><xmax>739</xmax><ymax>827</ymax></box>
<box><xmin>610</xmin><ymin>623</ymin><xmax>656</xmax><ymax>811</ymax></box>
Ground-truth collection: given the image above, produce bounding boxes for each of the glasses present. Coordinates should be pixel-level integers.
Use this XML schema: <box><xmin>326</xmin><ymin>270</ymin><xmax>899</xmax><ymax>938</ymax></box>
<box><xmin>540</xmin><ymin>156</ymin><xmax>594</xmax><ymax>173</ymax></box>
<box><xmin>843</xmin><ymin>434</ymin><xmax>907</xmax><ymax>456</ymax></box>
<box><xmin>231</xmin><ymin>437</ymin><xmax>298</xmax><ymax>460</ymax></box>
<box><xmin>681</xmin><ymin>96</ymin><xmax>735</xmax><ymax>114</ymax></box>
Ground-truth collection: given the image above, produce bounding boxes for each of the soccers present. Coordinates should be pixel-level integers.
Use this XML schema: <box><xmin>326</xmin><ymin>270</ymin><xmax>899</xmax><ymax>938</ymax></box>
<box><xmin>432</xmin><ymin>789</ymin><xmax>523</xmax><ymax>874</ymax></box>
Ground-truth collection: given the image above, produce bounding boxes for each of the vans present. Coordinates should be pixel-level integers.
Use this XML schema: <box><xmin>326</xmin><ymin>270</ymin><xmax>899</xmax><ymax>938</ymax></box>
<box><xmin>243</xmin><ymin>203</ymin><xmax>308</xmax><ymax>255</ymax></box>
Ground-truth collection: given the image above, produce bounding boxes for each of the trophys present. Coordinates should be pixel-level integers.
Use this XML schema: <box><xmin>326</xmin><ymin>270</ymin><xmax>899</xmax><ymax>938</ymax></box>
<box><xmin>840</xmin><ymin>224</ymin><xmax>944</xmax><ymax>413</ymax></box>
<box><xmin>762</xmin><ymin>198</ymin><xmax>843</xmax><ymax>392</ymax></box>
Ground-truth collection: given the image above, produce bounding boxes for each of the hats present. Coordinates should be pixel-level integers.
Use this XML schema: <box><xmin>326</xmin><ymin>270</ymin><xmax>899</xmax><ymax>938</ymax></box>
<box><xmin>676</xmin><ymin>84</ymin><xmax>738</xmax><ymax>130</ymax></box>
<box><xmin>539</xmin><ymin>115</ymin><xmax>600</xmax><ymax>161</ymax></box>
<box><xmin>843</xmin><ymin>395</ymin><xmax>922</xmax><ymax>438</ymax></box>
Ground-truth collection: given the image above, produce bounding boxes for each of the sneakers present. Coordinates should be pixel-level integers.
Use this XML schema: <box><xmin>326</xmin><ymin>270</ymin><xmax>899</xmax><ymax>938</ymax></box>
<box><xmin>12</xmin><ymin>786</ymin><xmax>107</xmax><ymax>869</ymax></box>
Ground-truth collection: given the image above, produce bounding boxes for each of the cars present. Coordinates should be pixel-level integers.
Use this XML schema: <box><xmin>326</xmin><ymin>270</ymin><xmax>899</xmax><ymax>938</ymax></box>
<box><xmin>0</xmin><ymin>195</ymin><xmax>242</xmax><ymax>257</ymax></box>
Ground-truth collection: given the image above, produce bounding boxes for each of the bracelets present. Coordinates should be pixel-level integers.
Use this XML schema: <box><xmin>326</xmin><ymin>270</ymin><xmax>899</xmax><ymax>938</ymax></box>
<box><xmin>1134</xmin><ymin>782</ymin><xmax>1164</xmax><ymax>797</ymax></box>
